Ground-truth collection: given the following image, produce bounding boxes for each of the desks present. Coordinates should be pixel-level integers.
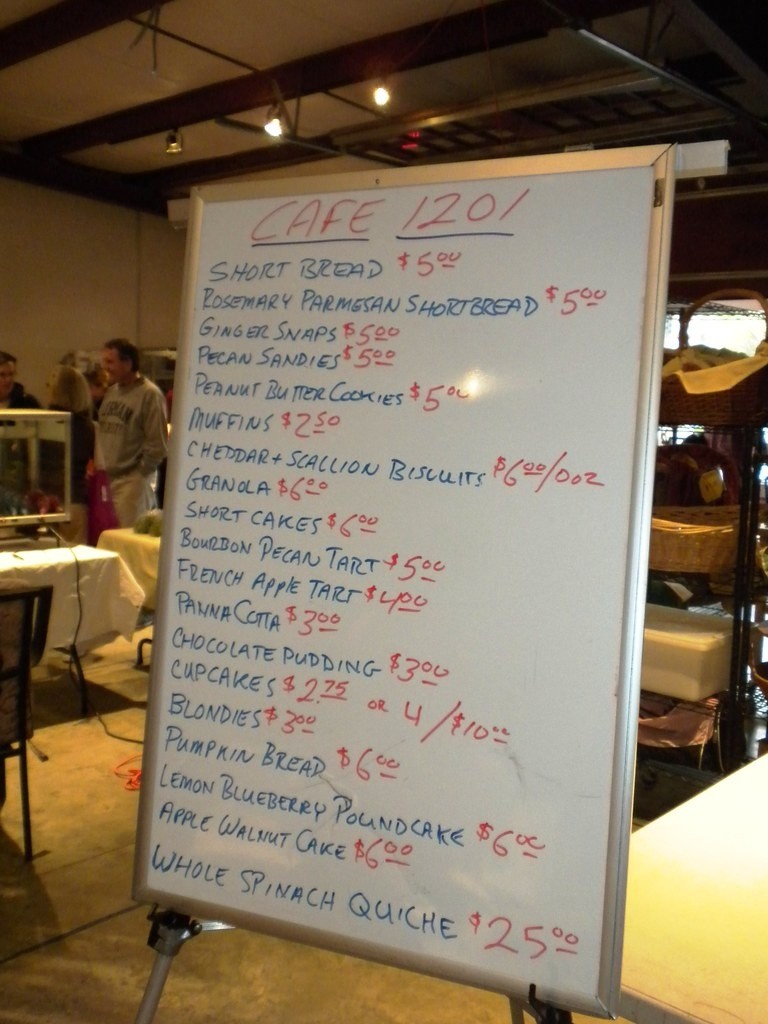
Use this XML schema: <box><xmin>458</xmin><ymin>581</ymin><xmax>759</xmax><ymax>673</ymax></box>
<box><xmin>617</xmin><ymin>750</ymin><xmax>768</xmax><ymax>1024</ymax></box>
<box><xmin>0</xmin><ymin>535</ymin><xmax>120</xmax><ymax>716</ymax></box>
<box><xmin>102</xmin><ymin>527</ymin><xmax>162</xmax><ymax>670</ymax></box>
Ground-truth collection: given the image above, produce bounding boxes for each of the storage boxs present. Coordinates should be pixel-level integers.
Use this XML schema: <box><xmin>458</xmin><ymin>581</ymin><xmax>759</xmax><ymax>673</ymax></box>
<box><xmin>631</xmin><ymin>759</ymin><xmax>723</xmax><ymax>834</ymax></box>
<box><xmin>640</xmin><ymin>604</ymin><xmax>735</xmax><ymax>702</ymax></box>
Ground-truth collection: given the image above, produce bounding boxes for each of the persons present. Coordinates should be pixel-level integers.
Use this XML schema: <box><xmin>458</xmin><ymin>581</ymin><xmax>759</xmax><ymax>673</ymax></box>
<box><xmin>0</xmin><ymin>351</ymin><xmax>47</xmax><ymax>454</ymax></box>
<box><xmin>72</xmin><ymin>354</ymin><xmax>110</xmax><ymax>402</ymax></box>
<box><xmin>93</xmin><ymin>338</ymin><xmax>168</xmax><ymax>531</ymax></box>
<box><xmin>22</xmin><ymin>363</ymin><xmax>96</xmax><ymax>547</ymax></box>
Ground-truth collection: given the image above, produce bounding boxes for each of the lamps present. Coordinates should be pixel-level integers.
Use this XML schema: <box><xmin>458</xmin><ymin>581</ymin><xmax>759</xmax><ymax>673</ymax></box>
<box><xmin>164</xmin><ymin>127</ymin><xmax>184</xmax><ymax>153</ymax></box>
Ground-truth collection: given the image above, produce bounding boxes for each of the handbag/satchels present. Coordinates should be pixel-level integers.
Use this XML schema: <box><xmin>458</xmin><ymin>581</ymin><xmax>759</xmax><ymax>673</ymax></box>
<box><xmin>87</xmin><ymin>469</ymin><xmax>121</xmax><ymax>547</ymax></box>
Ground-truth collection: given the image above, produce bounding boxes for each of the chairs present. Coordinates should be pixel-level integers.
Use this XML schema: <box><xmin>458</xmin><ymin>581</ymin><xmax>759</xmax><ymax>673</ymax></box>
<box><xmin>0</xmin><ymin>584</ymin><xmax>53</xmax><ymax>861</ymax></box>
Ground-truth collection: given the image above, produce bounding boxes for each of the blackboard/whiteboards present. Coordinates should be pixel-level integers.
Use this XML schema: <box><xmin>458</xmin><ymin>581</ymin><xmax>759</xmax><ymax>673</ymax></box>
<box><xmin>128</xmin><ymin>143</ymin><xmax>681</xmax><ymax>1021</ymax></box>
